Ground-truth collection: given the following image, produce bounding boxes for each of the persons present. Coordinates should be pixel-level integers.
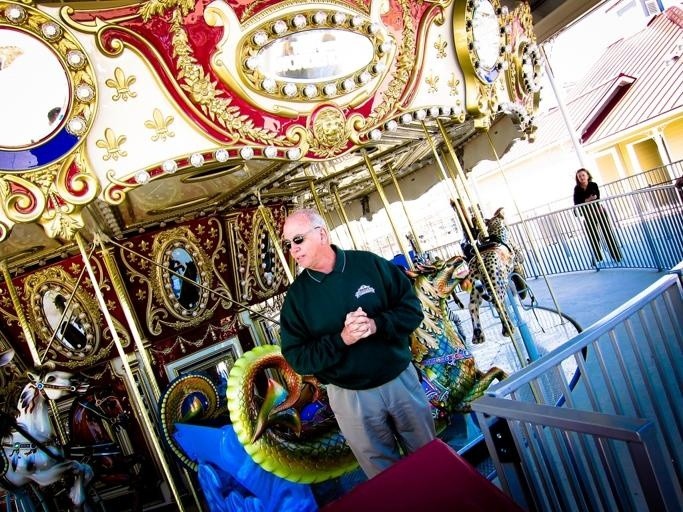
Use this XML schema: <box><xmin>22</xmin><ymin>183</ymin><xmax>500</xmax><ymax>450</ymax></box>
<box><xmin>572</xmin><ymin>168</ymin><xmax>625</xmax><ymax>270</ymax></box>
<box><xmin>280</xmin><ymin>208</ymin><xmax>438</xmax><ymax>480</ymax></box>
<box><xmin>0</xmin><ymin>1</ymin><xmax>547</xmax><ymax>511</ymax></box>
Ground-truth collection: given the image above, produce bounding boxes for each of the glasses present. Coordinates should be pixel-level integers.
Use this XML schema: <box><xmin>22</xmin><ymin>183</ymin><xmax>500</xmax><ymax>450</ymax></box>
<box><xmin>284</xmin><ymin>226</ymin><xmax>322</xmax><ymax>250</ymax></box>
